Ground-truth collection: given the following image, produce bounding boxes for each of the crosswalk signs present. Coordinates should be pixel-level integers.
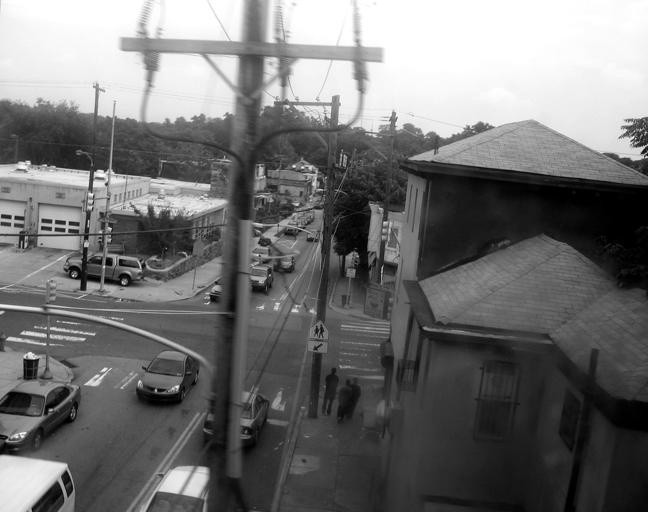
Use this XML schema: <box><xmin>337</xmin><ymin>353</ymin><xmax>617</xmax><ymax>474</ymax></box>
<box><xmin>308</xmin><ymin>320</ymin><xmax>329</xmax><ymax>339</ymax></box>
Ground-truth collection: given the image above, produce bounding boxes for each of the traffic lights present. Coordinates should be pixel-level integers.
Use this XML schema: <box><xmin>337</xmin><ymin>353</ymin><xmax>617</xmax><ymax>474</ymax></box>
<box><xmin>381</xmin><ymin>221</ymin><xmax>389</xmax><ymax>242</ymax></box>
<box><xmin>46</xmin><ymin>280</ymin><xmax>57</xmax><ymax>303</ymax></box>
<box><xmin>108</xmin><ymin>227</ymin><xmax>112</xmax><ymax>244</ymax></box>
<box><xmin>83</xmin><ymin>191</ymin><xmax>94</xmax><ymax>212</ymax></box>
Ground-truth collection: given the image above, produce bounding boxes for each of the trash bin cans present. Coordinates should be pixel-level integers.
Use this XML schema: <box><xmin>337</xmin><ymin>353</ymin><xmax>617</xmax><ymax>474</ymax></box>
<box><xmin>23</xmin><ymin>351</ymin><xmax>39</xmax><ymax>379</ymax></box>
<box><xmin>342</xmin><ymin>295</ymin><xmax>351</xmax><ymax>305</ymax></box>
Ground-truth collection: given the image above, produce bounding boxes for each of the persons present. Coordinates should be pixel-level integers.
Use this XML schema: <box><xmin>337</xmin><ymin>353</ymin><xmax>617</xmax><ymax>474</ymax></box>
<box><xmin>18</xmin><ymin>229</ymin><xmax>27</xmax><ymax>249</ymax></box>
<box><xmin>322</xmin><ymin>367</ymin><xmax>361</xmax><ymax>424</ymax></box>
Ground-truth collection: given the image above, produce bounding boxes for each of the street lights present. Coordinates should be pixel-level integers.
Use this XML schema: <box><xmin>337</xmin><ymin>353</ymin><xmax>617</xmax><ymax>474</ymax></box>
<box><xmin>75</xmin><ymin>150</ymin><xmax>95</xmax><ymax>291</ymax></box>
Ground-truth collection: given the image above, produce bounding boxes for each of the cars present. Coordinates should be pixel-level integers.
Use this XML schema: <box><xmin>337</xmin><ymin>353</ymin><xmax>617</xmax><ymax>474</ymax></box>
<box><xmin>307</xmin><ymin>229</ymin><xmax>320</xmax><ymax>242</ymax></box>
<box><xmin>314</xmin><ymin>199</ymin><xmax>324</xmax><ymax>209</ymax></box>
<box><xmin>284</xmin><ymin>208</ymin><xmax>315</xmax><ymax>234</ymax></box>
<box><xmin>209</xmin><ymin>246</ymin><xmax>296</xmax><ymax>302</ymax></box>
<box><xmin>0</xmin><ymin>379</ymin><xmax>81</xmax><ymax>512</ymax></box>
<box><xmin>142</xmin><ymin>391</ymin><xmax>269</xmax><ymax>512</ymax></box>
<box><xmin>136</xmin><ymin>350</ymin><xmax>199</xmax><ymax>402</ymax></box>
<box><xmin>63</xmin><ymin>252</ymin><xmax>146</xmax><ymax>286</ymax></box>
